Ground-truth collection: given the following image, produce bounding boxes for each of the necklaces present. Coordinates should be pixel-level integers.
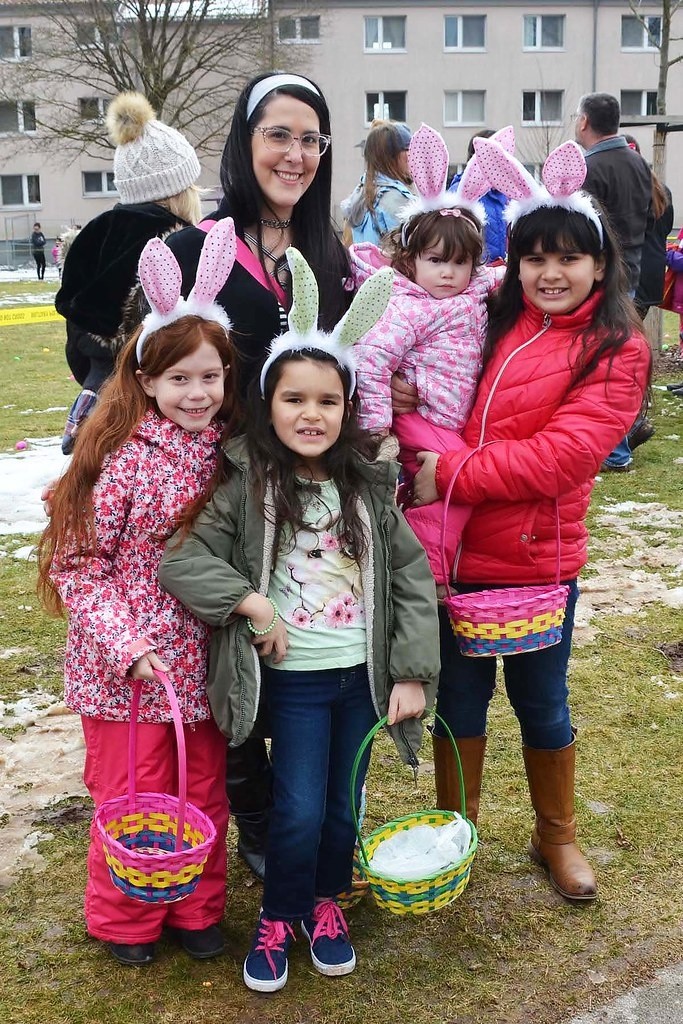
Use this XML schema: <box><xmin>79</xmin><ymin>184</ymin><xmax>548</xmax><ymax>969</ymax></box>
<box><xmin>260</xmin><ymin>217</ymin><xmax>291</xmax><ymax>229</ymax></box>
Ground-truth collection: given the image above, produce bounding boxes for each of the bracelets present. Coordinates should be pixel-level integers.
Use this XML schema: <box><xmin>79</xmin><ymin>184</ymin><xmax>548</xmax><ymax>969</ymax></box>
<box><xmin>247</xmin><ymin>597</ymin><xmax>278</xmax><ymax>635</ymax></box>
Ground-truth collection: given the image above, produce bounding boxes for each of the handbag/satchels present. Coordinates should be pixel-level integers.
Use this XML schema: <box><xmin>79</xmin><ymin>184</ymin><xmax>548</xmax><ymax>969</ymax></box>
<box><xmin>659</xmin><ymin>244</ymin><xmax>679</xmax><ymax>311</ymax></box>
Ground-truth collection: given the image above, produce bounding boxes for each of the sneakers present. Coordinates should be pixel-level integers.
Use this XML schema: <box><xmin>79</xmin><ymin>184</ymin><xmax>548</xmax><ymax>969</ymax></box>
<box><xmin>244</xmin><ymin>913</ymin><xmax>297</xmax><ymax>992</ymax></box>
<box><xmin>628</xmin><ymin>421</ymin><xmax>655</xmax><ymax>450</ymax></box>
<box><xmin>300</xmin><ymin>901</ymin><xmax>356</xmax><ymax>976</ymax></box>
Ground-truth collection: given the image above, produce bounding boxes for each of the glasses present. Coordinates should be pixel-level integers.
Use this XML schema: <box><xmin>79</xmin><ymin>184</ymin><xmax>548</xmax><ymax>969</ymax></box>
<box><xmin>252</xmin><ymin>125</ymin><xmax>331</xmax><ymax>157</ymax></box>
<box><xmin>571</xmin><ymin>113</ymin><xmax>582</xmax><ymax>121</ymax></box>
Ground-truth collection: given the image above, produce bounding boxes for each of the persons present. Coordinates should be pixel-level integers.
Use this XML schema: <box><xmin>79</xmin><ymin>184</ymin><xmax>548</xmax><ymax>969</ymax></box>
<box><xmin>60</xmin><ymin>72</ymin><xmax>360</xmax><ymax>455</ymax></box>
<box><xmin>571</xmin><ymin>92</ymin><xmax>651</xmax><ymax>474</ymax></box>
<box><xmin>57</xmin><ymin>89</ymin><xmax>202</xmax><ymax>385</ymax></box>
<box><xmin>391</xmin><ymin>193</ymin><xmax>653</xmax><ymax>902</ymax></box>
<box><xmin>30</xmin><ymin>222</ymin><xmax>46</xmax><ymax>280</ymax></box>
<box><xmin>52</xmin><ymin>238</ymin><xmax>61</xmax><ymax>277</ymax></box>
<box><xmin>621</xmin><ymin>133</ymin><xmax>674</xmax><ymax>322</ymax></box>
<box><xmin>349</xmin><ymin>205</ymin><xmax>496</xmax><ymax>606</ymax></box>
<box><xmin>157</xmin><ymin>345</ymin><xmax>440</xmax><ymax>993</ymax></box>
<box><xmin>448</xmin><ymin>129</ymin><xmax>507</xmax><ymax>264</ymax></box>
<box><xmin>38</xmin><ymin>310</ymin><xmax>238</xmax><ymax>965</ymax></box>
<box><xmin>340</xmin><ymin>118</ymin><xmax>416</xmax><ymax>243</ymax></box>
<box><xmin>666</xmin><ymin>228</ymin><xmax>683</xmax><ymax>395</ymax></box>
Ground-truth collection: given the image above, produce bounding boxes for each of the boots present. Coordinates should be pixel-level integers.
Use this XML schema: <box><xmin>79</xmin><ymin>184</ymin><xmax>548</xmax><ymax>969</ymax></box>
<box><xmin>524</xmin><ymin>726</ymin><xmax>599</xmax><ymax>900</ymax></box>
<box><xmin>428</xmin><ymin>722</ymin><xmax>488</xmax><ymax>825</ymax></box>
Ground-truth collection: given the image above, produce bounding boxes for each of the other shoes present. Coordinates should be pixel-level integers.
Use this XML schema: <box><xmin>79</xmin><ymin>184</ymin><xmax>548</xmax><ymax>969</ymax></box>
<box><xmin>672</xmin><ymin>386</ymin><xmax>683</xmax><ymax>395</ymax></box>
<box><xmin>236</xmin><ymin>834</ymin><xmax>265</xmax><ymax>879</ymax></box>
<box><xmin>667</xmin><ymin>381</ymin><xmax>683</xmax><ymax>391</ymax></box>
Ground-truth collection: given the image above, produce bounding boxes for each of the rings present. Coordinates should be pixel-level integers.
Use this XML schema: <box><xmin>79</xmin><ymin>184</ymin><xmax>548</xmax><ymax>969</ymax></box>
<box><xmin>408</xmin><ymin>490</ymin><xmax>411</xmax><ymax>495</ymax></box>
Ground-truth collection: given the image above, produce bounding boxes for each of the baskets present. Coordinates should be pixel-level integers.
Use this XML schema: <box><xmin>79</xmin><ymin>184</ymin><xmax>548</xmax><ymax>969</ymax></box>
<box><xmin>350</xmin><ymin>708</ymin><xmax>478</xmax><ymax>914</ymax></box>
<box><xmin>336</xmin><ymin>845</ymin><xmax>369</xmax><ymax>909</ymax></box>
<box><xmin>96</xmin><ymin>668</ymin><xmax>216</xmax><ymax>905</ymax></box>
<box><xmin>437</xmin><ymin>439</ymin><xmax>570</xmax><ymax>657</ymax></box>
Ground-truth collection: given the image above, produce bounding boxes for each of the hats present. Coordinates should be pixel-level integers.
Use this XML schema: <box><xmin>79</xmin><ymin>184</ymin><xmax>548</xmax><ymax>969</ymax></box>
<box><xmin>108</xmin><ymin>96</ymin><xmax>202</xmax><ymax>206</ymax></box>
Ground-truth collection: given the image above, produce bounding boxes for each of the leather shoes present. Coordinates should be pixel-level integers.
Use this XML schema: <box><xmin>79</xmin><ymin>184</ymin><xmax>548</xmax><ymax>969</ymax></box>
<box><xmin>109</xmin><ymin>942</ymin><xmax>154</xmax><ymax>965</ymax></box>
<box><xmin>177</xmin><ymin>924</ymin><xmax>227</xmax><ymax>958</ymax></box>
